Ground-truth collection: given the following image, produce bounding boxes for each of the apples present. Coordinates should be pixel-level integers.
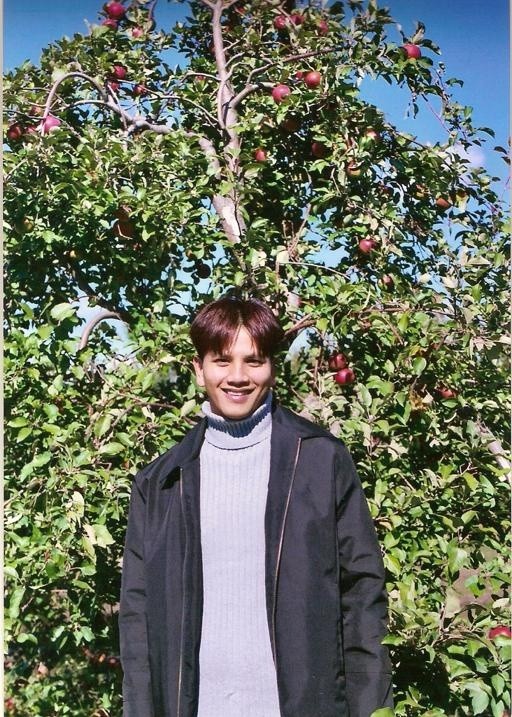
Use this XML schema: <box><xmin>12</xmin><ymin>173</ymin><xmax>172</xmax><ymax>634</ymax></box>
<box><xmin>413</xmin><ymin>182</ymin><xmax>425</xmax><ymax>199</ymax></box>
<box><xmin>195</xmin><ymin>75</ymin><xmax>204</xmax><ymax>80</ymax></box>
<box><xmin>296</xmin><ymin>70</ymin><xmax>320</xmax><ymax>87</ymax></box>
<box><xmin>376</xmin><ymin>186</ymin><xmax>393</xmax><ymax>202</ymax></box>
<box><xmin>114</xmin><ymin>205</ymin><xmax>137</xmax><ymax>240</ymax></box>
<box><xmin>329</xmin><ymin>354</ymin><xmax>355</xmax><ymax>383</ymax></box>
<box><xmin>274</xmin><ymin>15</ymin><xmax>327</xmax><ymax>34</ymax></box>
<box><xmin>197</xmin><ymin>264</ymin><xmax>211</xmax><ymax>279</ymax></box>
<box><xmin>132</xmin><ymin>29</ymin><xmax>142</xmax><ymax>37</ymax></box>
<box><xmin>108</xmin><ymin>66</ymin><xmax>125</xmax><ymax>90</ymax></box>
<box><xmin>134</xmin><ymin>85</ymin><xmax>148</xmax><ymax>95</ymax></box>
<box><xmin>441</xmin><ymin>387</ymin><xmax>458</xmax><ymax>399</ymax></box>
<box><xmin>312</xmin><ymin>129</ymin><xmax>380</xmax><ymax>178</ymax></box>
<box><xmin>255</xmin><ymin>150</ymin><xmax>265</xmax><ymax>161</ymax></box>
<box><xmin>285</xmin><ymin>119</ymin><xmax>297</xmax><ymax>130</ymax></box>
<box><xmin>489</xmin><ymin>626</ymin><xmax>512</xmax><ymax>639</ymax></box>
<box><xmin>359</xmin><ymin>239</ymin><xmax>378</xmax><ymax>257</ymax></box>
<box><xmin>379</xmin><ymin>275</ymin><xmax>394</xmax><ymax>292</ymax></box>
<box><xmin>403</xmin><ymin>45</ymin><xmax>421</xmax><ymax>60</ymax></box>
<box><xmin>104</xmin><ymin>3</ymin><xmax>125</xmax><ymax>27</ymax></box>
<box><xmin>272</xmin><ymin>85</ymin><xmax>290</xmax><ymax>104</ymax></box>
<box><xmin>436</xmin><ymin>198</ymin><xmax>450</xmax><ymax>209</ymax></box>
<box><xmin>8</xmin><ymin>106</ymin><xmax>62</xmax><ymax>140</ymax></box>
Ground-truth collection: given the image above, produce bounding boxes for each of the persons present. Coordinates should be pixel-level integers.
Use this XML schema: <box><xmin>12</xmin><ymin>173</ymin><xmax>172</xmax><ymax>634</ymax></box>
<box><xmin>117</xmin><ymin>294</ymin><xmax>401</xmax><ymax>717</ymax></box>
